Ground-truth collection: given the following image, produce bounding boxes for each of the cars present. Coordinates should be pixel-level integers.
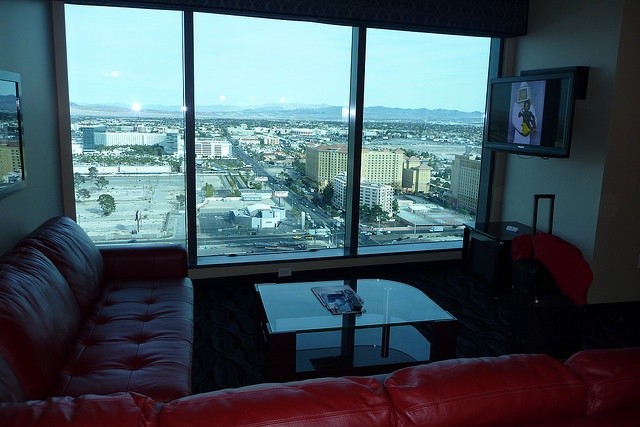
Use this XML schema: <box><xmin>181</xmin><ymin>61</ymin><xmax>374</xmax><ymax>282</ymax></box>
<box><xmin>304</xmin><ymin>235</ymin><xmax>313</xmax><ymax>240</ymax></box>
<box><xmin>364</xmin><ymin>231</ymin><xmax>373</xmax><ymax>236</ymax></box>
<box><xmin>292</xmin><ymin>235</ymin><xmax>302</xmax><ymax>240</ymax></box>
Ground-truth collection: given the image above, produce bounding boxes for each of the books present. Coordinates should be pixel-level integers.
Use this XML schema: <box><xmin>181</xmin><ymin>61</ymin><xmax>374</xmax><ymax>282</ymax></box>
<box><xmin>318</xmin><ymin>290</ymin><xmax>362</xmax><ymax>314</ymax></box>
<box><xmin>311</xmin><ymin>285</ymin><xmax>364</xmax><ymax>307</ymax></box>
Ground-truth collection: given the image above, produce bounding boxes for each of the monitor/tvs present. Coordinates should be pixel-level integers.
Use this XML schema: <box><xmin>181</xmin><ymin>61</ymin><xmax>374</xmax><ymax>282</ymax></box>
<box><xmin>0</xmin><ymin>70</ymin><xmax>28</xmax><ymax>200</ymax></box>
<box><xmin>483</xmin><ymin>72</ymin><xmax>578</xmax><ymax>159</ymax></box>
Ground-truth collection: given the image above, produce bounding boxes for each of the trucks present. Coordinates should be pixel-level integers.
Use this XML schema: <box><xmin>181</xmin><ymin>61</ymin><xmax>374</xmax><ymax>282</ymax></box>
<box><xmin>429</xmin><ymin>225</ymin><xmax>444</xmax><ymax>232</ymax></box>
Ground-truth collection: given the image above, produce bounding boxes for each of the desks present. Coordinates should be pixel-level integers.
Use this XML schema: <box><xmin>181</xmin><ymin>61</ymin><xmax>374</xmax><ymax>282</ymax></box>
<box><xmin>456</xmin><ymin>222</ymin><xmax>547</xmax><ymax>314</ymax></box>
<box><xmin>250</xmin><ymin>277</ymin><xmax>457</xmax><ymax>385</ymax></box>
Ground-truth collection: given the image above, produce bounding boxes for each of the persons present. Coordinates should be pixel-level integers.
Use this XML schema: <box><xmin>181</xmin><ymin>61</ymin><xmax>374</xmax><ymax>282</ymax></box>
<box><xmin>511</xmin><ymin>81</ymin><xmax>536</xmax><ymax>144</ymax></box>
<box><xmin>516</xmin><ymin>100</ymin><xmax>537</xmax><ymax>137</ymax></box>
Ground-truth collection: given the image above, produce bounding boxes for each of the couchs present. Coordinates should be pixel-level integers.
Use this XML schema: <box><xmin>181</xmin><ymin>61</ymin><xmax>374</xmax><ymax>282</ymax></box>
<box><xmin>1</xmin><ymin>213</ymin><xmax>195</xmax><ymax>402</ymax></box>
<box><xmin>0</xmin><ymin>347</ymin><xmax>640</xmax><ymax>427</ymax></box>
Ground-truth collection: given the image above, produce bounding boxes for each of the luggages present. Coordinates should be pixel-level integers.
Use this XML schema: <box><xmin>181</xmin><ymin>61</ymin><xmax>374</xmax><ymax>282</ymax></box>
<box><xmin>509</xmin><ymin>194</ymin><xmax>561</xmax><ymax>354</ymax></box>
